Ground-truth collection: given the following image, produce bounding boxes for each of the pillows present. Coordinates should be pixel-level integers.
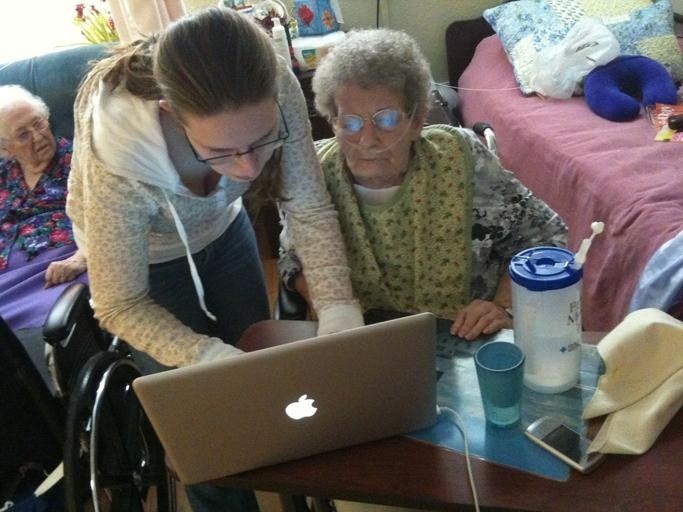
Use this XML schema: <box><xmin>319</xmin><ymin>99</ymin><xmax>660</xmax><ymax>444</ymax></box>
<box><xmin>481</xmin><ymin>0</ymin><xmax>682</xmax><ymax>101</ymax></box>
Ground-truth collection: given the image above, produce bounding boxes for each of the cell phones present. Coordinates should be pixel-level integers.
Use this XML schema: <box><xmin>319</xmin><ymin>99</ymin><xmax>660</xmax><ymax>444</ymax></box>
<box><xmin>522</xmin><ymin>415</ymin><xmax>609</xmax><ymax>476</ymax></box>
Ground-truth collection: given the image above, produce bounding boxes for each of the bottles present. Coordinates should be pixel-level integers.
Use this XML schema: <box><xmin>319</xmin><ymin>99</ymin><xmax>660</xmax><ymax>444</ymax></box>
<box><xmin>507</xmin><ymin>220</ymin><xmax>605</xmax><ymax>395</ymax></box>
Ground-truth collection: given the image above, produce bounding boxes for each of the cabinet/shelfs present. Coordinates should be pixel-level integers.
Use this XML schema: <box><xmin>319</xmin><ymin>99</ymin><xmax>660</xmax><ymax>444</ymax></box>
<box><xmin>264</xmin><ymin>70</ymin><xmax>335</xmax><ymax>259</ymax></box>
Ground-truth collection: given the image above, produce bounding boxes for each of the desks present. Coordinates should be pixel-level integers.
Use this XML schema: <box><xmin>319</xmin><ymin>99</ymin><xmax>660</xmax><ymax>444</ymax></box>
<box><xmin>172</xmin><ymin>318</ymin><xmax>683</xmax><ymax>512</ymax></box>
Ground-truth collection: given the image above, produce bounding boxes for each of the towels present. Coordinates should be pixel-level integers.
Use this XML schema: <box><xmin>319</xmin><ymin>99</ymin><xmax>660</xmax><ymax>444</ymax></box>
<box><xmin>582</xmin><ymin>308</ymin><xmax>683</xmax><ymax>456</ymax></box>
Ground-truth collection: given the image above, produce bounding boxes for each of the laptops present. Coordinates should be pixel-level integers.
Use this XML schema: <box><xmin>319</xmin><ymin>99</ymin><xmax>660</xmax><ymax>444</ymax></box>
<box><xmin>128</xmin><ymin>312</ymin><xmax>439</xmax><ymax>487</ymax></box>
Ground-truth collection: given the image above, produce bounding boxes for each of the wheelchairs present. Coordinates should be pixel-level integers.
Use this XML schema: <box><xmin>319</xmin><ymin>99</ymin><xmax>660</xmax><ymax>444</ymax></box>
<box><xmin>0</xmin><ymin>263</ymin><xmax>179</xmax><ymax>511</ymax></box>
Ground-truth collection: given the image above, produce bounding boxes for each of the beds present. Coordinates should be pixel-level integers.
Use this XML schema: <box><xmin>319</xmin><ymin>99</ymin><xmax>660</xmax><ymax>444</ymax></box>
<box><xmin>445</xmin><ymin>8</ymin><xmax>682</xmax><ymax>330</ymax></box>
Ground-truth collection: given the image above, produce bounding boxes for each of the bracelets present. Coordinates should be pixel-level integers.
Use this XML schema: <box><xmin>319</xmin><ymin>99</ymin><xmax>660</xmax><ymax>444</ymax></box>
<box><xmin>494</xmin><ymin>303</ymin><xmax>513</xmax><ymax>320</ymax></box>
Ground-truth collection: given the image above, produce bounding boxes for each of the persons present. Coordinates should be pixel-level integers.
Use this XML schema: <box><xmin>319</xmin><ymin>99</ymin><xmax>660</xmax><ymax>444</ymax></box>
<box><xmin>278</xmin><ymin>22</ymin><xmax>568</xmax><ymax>339</ymax></box>
<box><xmin>65</xmin><ymin>7</ymin><xmax>365</xmax><ymax>511</ymax></box>
<box><xmin>0</xmin><ymin>83</ymin><xmax>87</xmax><ymax>429</ymax></box>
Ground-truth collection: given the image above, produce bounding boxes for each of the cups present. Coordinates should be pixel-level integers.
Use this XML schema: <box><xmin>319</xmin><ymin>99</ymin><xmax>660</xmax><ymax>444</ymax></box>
<box><xmin>473</xmin><ymin>340</ymin><xmax>525</xmax><ymax>426</ymax></box>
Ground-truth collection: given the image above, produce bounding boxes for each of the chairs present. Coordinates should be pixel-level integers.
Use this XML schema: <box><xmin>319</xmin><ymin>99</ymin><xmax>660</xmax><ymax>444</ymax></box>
<box><xmin>0</xmin><ymin>44</ymin><xmax>117</xmax><ymax>140</ymax></box>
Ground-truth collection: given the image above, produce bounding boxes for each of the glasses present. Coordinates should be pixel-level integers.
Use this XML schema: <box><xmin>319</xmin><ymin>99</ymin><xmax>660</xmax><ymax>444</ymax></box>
<box><xmin>329</xmin><ymin>104</ymin><xmax>411</xmax><ymax>137</ymax></box>
<box><xmin>2</xmin><ymin>115</ymin><xmax>49</xmax><ymax>149</ymax></box>
<box><xmin>179</xmin><ymin>99</ymin><xmax>289</xmax><ymax>164</ymax></box>
